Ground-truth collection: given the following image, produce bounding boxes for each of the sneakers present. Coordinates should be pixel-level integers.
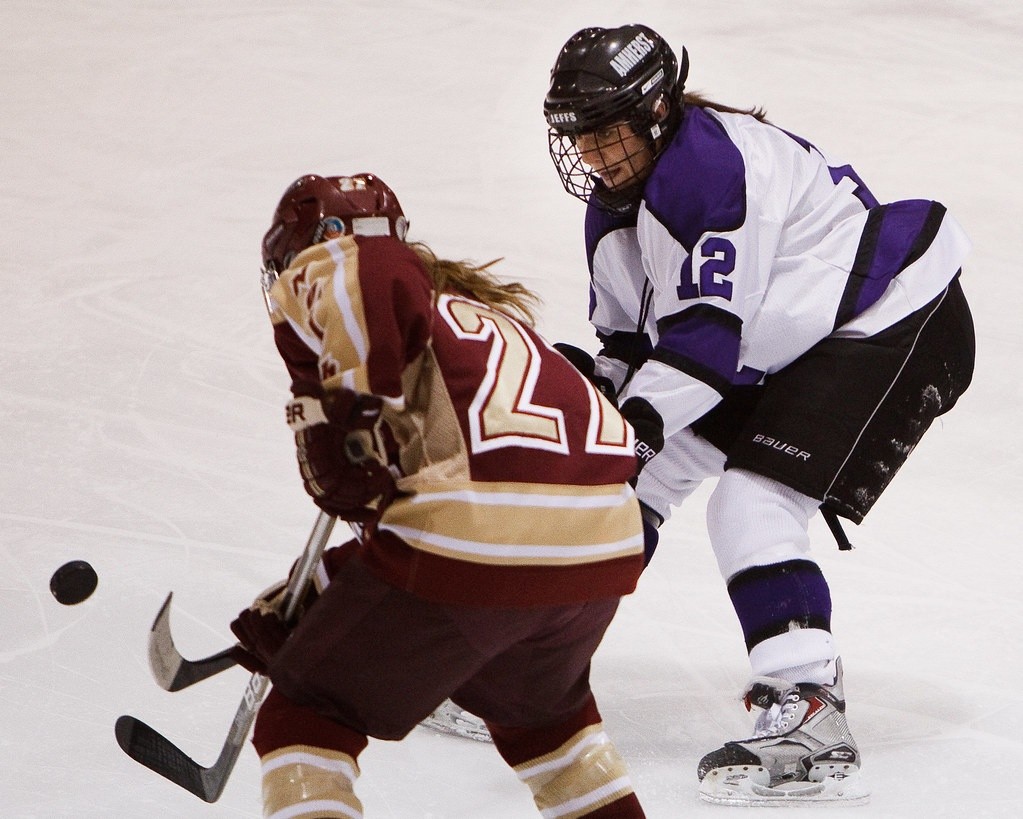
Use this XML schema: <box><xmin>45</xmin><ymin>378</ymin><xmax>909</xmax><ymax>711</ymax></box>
<box><xmin>417</xmin><ymin>696</ymin><xmax>493</xmax><ymax>744</ymax></box>
<box><xmin>697</xmin><ymin>654</ymin><xmax>871</xmax><ymax>806</ymax></box>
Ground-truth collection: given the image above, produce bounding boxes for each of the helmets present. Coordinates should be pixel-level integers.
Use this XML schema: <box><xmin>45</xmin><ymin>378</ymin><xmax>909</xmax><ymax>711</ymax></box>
<box><xmin>261</xmin><ymin>173</ymin><xmax>409</xmax><ymax>275</ymax></box>
<box><xmin>541</xmin><ymin>25</ymin><xmax>678</xmax><ymax>218</ymax></box>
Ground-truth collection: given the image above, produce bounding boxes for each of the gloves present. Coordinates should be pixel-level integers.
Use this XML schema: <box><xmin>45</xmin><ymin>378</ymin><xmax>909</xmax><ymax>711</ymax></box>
<box><xmin>614</xmin><ymin>396</ymin><xmax>665</xmax><ymax>492</ymax></box>
<box><xmin>552</xmin><ymin>343</ymin><xmax>619</xmax><ymax>412</ymax></box>
<box><xmin>231</xmin><ymin>555</ymin><xmax>323</xmax><ymax>660</ymax></box>
<box><xmin>282</xmin><ymin>387</ymin><xmax>405</xmax><ymax>526</ymax></box>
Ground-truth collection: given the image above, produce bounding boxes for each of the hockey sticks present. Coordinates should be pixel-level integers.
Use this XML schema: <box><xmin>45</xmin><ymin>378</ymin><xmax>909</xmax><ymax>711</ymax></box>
<box><xmin>147</xmin><ymin>587</ymin><xmax>239</xmax><ymax>694</ymax></box>
<box><xmin>114</xmin><ymin>393</ymin><xmax>386</xmax><ymax>804</ymax></box>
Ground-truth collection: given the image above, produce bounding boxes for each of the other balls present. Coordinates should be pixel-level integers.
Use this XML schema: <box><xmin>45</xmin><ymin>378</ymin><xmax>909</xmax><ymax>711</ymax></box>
<box><xmin>49</xmin><ymin>560</ymin><xmax>99</xmax><ymax>606</ymax></box>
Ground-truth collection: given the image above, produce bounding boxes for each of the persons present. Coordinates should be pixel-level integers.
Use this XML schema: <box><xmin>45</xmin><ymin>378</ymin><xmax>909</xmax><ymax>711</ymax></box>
<box><xmin>231</xmin><ymin>173</ymin><xmax>646</xmax><ymax>819</ymax></box>
<box><xmin>421</xmin><ymin>24</ymin><xmax>974</xmax><ymax>801</ymax></box>
